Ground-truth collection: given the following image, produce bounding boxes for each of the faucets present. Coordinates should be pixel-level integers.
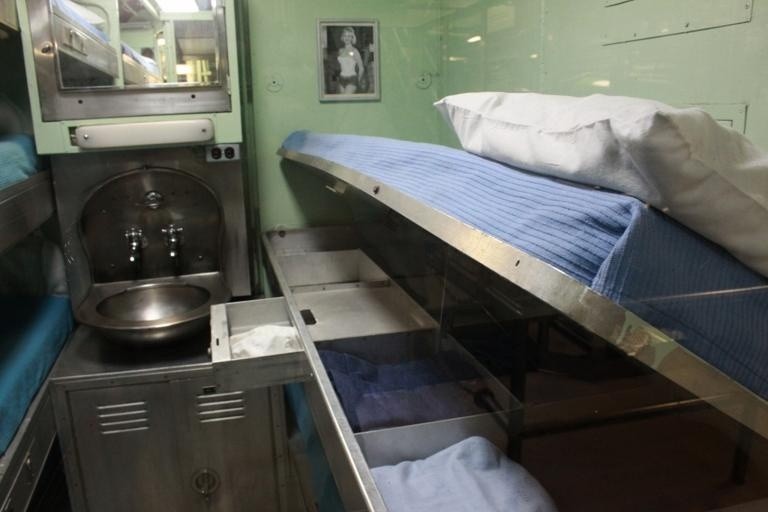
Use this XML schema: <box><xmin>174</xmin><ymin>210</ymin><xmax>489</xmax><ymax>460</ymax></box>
<box><xmin>160</xmin><ymin>225</ymin><xmax>184</xmax><ymax>259</ymax></box>
<box><xmin>124</xmin><ymin>227</ymin><xmax>146</xmax><ymax>263</ymax></box>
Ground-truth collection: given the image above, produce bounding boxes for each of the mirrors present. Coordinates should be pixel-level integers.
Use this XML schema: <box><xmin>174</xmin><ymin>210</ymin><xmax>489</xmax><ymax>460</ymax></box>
<box><xmin>48</xmin><ymin>0</ymin><xmax>225</xmax><ymax>90</ymax></box>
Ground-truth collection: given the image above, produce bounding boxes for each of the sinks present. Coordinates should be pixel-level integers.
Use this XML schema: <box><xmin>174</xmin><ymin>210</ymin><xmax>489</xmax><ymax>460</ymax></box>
<box><xmin>76</xmin><ymin>272</ymin><xmax>232</xmax><ymax>346</ymax></box>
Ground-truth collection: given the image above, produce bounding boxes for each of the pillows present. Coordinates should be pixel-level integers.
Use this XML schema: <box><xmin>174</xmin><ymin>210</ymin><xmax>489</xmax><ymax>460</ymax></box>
<box><xmin>430</xmin><ymin>91</ymin><xmax>768</xmax><ymax>282</ymax></box>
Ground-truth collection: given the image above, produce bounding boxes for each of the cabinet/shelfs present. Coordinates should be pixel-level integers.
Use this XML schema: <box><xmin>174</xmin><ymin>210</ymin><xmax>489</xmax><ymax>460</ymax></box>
<box><xmin>45</xmin><ymin>364</ymin><xmax>290</xmax><ymax>512</ymax></box>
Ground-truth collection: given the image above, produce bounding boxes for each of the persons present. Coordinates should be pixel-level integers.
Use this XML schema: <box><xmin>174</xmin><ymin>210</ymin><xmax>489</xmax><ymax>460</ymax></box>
<box><xmin>336</xmin><ymin>26</ymin><xmax>366</xmax><ymax>95</ymax></box>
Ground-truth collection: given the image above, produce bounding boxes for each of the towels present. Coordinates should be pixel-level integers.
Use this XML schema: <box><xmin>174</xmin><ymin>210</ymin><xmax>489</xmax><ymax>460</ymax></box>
<box><xmin>232</xmin><ymin>322</ymin><xmax>305</xmax><ymax>358</ymax></box>
<box><xmin>356</xmin><ymin>434</ymin><xmax>558</xmax><ymax>512</ymax></box>
<box><xmin>316</xmin><ymin>340</ymin><xmax>494</xmax><ymax>434</ymax></box>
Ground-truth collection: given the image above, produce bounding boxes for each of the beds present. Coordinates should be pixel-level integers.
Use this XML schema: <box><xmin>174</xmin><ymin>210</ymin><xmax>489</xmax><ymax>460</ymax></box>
<box><xmin>265</xmin><ymin>102</ymin><xmax>767</xmax><ymax>512</ymax></box>
<box><xmin>0</xmin><ymin>0</ymin><xmax>73</xmax><ymax>512</ymax></box>
<box><xmin>50</xmin><ymin>0</ymin><xmax>119</xmax><ymax>87</ymax></box>
<box><xmin>114</xmin><ymin>0</ymin><xmax>165</xmax><ymax>85</ymax></box>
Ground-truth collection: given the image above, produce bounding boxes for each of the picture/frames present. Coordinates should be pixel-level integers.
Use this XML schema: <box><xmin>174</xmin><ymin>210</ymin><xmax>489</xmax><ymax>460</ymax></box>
<box><xmin>317</xmin><ymin>17</ymin><xmax>381</xmax><ymax>101</ymax></box>
